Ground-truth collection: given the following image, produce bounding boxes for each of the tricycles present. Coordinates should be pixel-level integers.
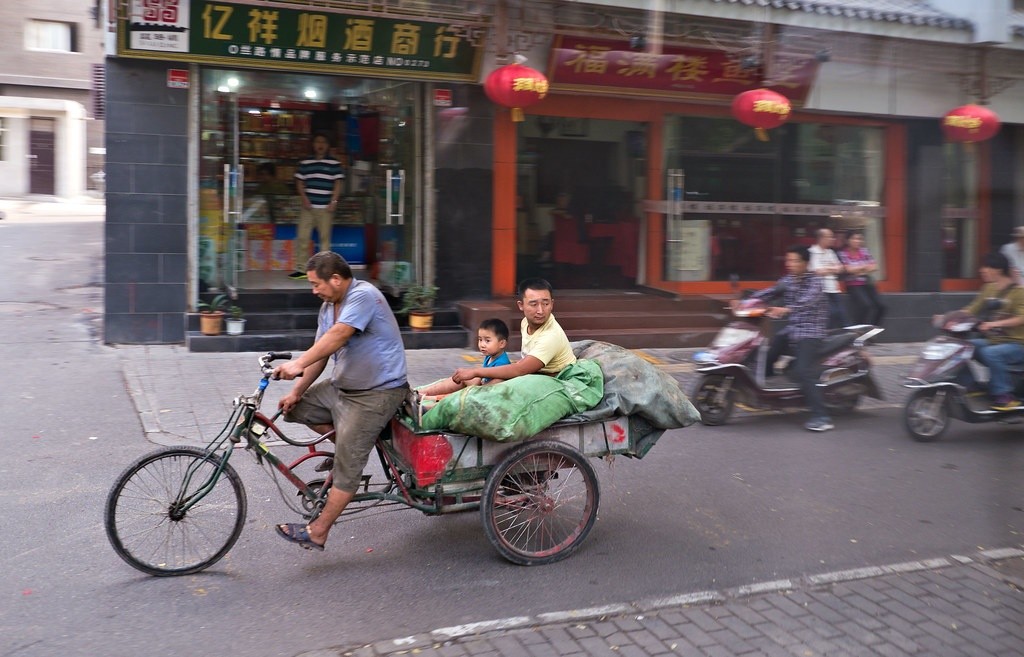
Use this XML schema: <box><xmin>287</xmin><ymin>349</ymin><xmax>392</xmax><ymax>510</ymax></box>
<box><xmin>103</xmin><ymin>350</ymin><xmax>634</xmax><ymax>576</ymax></box>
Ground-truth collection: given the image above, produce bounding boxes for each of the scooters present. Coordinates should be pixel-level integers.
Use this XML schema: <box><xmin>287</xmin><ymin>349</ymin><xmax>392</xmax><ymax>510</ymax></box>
<box><xmin>683</xmin><ymin>275</ymin><xmax>889</xmax><ymax>427</ymax></box>
<box><xmin>901</xmin><ymin>294</ymin><xmax>1023</xmax><ymax>444</ymax></box>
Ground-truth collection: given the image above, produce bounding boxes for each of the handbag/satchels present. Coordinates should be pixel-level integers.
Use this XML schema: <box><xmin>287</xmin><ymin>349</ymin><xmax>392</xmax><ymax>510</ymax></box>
<box><xmin>973</xmin><ymin>282</ymin><xmax>1018</xmax><ymax>338</ymax></box>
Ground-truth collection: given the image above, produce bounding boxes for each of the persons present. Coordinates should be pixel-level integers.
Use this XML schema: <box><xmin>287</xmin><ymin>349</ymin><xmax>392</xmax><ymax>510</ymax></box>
<box><xmin>728</xmin><ymin>247</ymin><xmax>833</xmax><ymax>431</ymax></box>
<box><xmin>402</xmin><ymin>278</ymin><xmax>576</xmax><ymax>426</ymax></box>
<box><xmin>288</xmin><ymin>133</ymin><xmax>344</xmax><ymax>279</ymax></box>
<box><xmin>270</xmin><ymin>250</ymin><xmax>408</xmax><ymax>549</ymax></box>
<box><xmin>838</xmin><ymin>231</ymin><xmax>886</xmax><ymax>324</ymax></box>
<box><xmin>534</xmin><ymin>177</ymin><xmax>632</xmax><ymax>274</ymax></box>
<box><xmin>256</xmin><ymin>161</ymin><xmax>275</xmax><ymax>193</ymax></box>
<box><xmin>807</xmin><ymin>228</ymin><xmax>851</xmax><ymax>327</ymax></box>
<box><xmin>932</xmin><ymin>226</ymin><xmax>1024</xmax><ymax>410</ymax></box>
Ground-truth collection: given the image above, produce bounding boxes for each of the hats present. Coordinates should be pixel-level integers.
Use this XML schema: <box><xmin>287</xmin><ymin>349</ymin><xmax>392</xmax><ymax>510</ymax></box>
<box><xmin>1008</xmin><ymin>226</ymin><xmax>1024</xmax><ymax>238</ymax></box>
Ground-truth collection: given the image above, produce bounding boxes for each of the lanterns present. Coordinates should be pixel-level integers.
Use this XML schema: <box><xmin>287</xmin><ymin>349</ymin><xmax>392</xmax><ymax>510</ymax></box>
<box><xmin>940</xmin><ymin>100</ymin><xmax>999</xmax><ymax>152</ymax></box>
<box><xmin>731</xmin><ymin>81</ymin><xmax>791</xmax><ymax>141</ymax></box>
<box><xmin>483</xmin><ymin>56</ymin><xmax>548</xmax><ymax>122</ymax></box>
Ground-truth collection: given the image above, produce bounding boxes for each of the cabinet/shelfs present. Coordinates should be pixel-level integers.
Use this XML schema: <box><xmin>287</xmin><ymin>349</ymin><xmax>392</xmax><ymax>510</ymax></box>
<box><xmin>201</xmin><ymin>95</ymin><xmax>315</xmax><ymax>178</ymax></box>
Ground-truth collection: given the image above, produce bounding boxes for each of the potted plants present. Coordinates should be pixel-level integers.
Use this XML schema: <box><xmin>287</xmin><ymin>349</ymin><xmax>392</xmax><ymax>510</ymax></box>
<box><xmin>396</xmin><ymin>285</ymin><xmax>440</xmax><ymax>333</ymax></box>
<box><xmin>224</xmin><ymin>305</ymin><xmax>247</xmax><ymax>335</ymax></box>
<box><xmin>195</xmin><ymin>293</ymin><xmax>229</xmax><ymax>334</ymax></box>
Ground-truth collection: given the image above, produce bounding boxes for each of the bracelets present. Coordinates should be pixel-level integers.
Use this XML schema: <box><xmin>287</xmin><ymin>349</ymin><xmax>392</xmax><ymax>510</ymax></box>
<box><xmin>332</xmin><ymin>200</ymin><xmax>338</xmax><ymax>202</ymax></box>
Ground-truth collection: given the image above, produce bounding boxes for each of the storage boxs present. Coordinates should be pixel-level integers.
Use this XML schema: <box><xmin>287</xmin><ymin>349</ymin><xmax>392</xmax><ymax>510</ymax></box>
<box><xmin>237</xmin><ymin>111</ymin><xmax>311</xmax><ymax>178</ymax></box>
<box><xmin>376</xmin><ymin>259</ymin><xmax>411</xmax><ymax>287</ymax></box>
<box><xmin>201</xmin><ymin>188</ymin><xmax>247</xmax><ymax>290</ymax></box>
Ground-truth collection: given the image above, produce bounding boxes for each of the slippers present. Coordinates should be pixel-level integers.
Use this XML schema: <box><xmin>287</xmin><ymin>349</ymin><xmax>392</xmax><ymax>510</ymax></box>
<box><xmin>315</xmin><ymin>456</ymin><xmax>333</xmax><ymax>472</ymax></box>
<box><xmin>275</xmin><ymin>522</ymin><xmax>325</xmax><ymax>551</ymax></box>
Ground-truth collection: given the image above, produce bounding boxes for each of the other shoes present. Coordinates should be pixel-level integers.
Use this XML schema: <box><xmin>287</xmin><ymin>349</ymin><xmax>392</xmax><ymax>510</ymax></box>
<box><xmin>286</xmin><ymin>271</ymin><xmax>308</xmax><ymax>278</ymax></box>
<box><xmin>407</xmin><ymin>389</ymin><xmax>423</xmax><ymax>406</ymax></box>
<box><xmin>990</xmin><ymin>397</ymin><xmax>1021</xmax><ymax>411</ymax></box>
<box><xmin>962</xmin><ymin>383</ymin><xmax>988</xmax><ymax>398</ymax></box>
<box><xmin>403</xmin><ymin>403</ymin><xmax>427</xmax><ymax>418</ymax></box>
<box><xmin>802</xmin><ymin>416</ymin><xmax>835</xmax><ymax>431</ymax></box>
